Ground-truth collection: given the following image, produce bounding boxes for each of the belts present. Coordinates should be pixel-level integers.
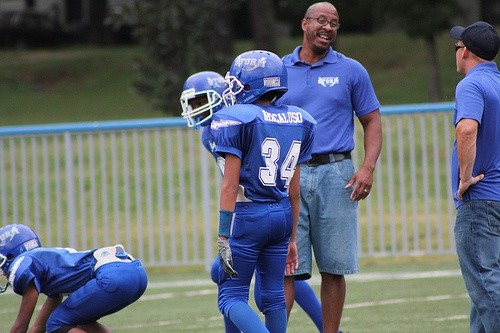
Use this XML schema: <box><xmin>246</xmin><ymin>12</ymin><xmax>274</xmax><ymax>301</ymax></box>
<box><xmin>300</xmin><ymin>152</ymin><xmax>352</xmax><ymax>167</ymax></box>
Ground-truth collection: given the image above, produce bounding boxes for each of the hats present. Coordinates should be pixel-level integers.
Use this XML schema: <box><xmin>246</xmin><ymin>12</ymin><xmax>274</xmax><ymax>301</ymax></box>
<box><xmin>449</xmin><ymin>21</ymin><xmax>500</xmax><ymax>61</ymax></box>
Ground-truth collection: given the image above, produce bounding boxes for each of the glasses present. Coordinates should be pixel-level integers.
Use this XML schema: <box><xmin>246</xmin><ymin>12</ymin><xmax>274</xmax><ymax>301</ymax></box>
<box><xmin>306</xmin><ymin>17</ymin><xmax>340</xmax><ymax>29</ymax></box>
<box><xmin>455</xmin><ymin>43</ymin><xmax>466</xmax><ymax>52</ymax></box>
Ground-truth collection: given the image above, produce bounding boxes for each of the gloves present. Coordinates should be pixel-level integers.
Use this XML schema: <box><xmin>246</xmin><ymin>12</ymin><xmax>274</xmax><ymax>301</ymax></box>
<box><xmin>217</xmin><ymin>238</ymin><xmax>239</xmax><ymax>278</ymax></box>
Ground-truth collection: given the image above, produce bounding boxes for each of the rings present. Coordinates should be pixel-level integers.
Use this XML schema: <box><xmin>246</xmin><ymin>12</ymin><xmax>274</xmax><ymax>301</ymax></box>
<box><xmin>364</xmin><ymin>189</ymin><xmax>367</xmax><ymax>192</ymax></box>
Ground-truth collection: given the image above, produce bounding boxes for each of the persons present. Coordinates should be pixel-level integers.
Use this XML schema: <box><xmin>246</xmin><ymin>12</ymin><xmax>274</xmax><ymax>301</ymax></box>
<box><xmin>180</xmin><ymin>1</ymin><xmax>383</xmax><ymax>332</ymax></box>
<box><xmin>449</xmin><ymin>22</ymin><xmax>500</xmax><ymax>333</ymax></box>
<box><xmin>0</xmin><ymin>224</ymin><xmax>148</xmax><ymax>333</ymax></box>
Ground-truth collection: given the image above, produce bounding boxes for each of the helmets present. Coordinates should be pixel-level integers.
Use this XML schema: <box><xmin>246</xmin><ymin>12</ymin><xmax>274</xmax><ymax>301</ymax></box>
<box><xmin>180</xmin><ymin>71</ymin><xmax>236</xmax><ymax>130</ymax></box>
<box><xmin>222</xmin><ymin>50</ymin><xmax>288</xmax><ymax>107</ymax></box>
<box><xmin>0</xmin><ymin>224</ymin><xmax>41</xmax><ymax>280</ymax></box>
